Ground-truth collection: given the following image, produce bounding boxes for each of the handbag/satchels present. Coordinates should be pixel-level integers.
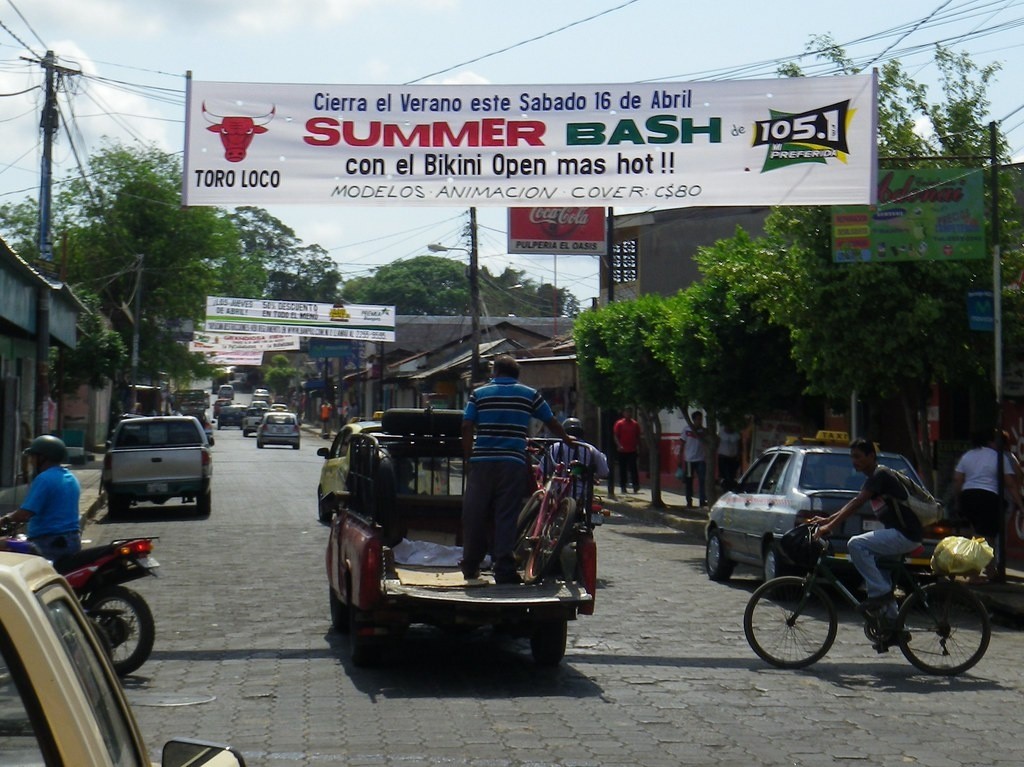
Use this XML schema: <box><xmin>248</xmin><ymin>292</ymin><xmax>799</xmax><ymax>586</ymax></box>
<box><xmin>781</xmin><ymin>524</ymin><xmax>834</xmax><ymax>571</ymax></box>
<box><xmin>929</xmin><ymin>535</ymin><xmax>995</xmax><ymax>576</ymax></box>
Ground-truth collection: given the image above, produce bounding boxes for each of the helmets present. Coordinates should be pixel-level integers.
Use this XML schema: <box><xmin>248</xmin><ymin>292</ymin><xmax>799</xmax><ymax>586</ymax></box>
<box><xmin>562</xmin><ymin>418</ymin><xmax>582</xmax><ymax>432</ymax></box>
<box><xmin>24</xmin><ymin>436</ymin><xmax>66</xmax><ymax>466</ymax></box>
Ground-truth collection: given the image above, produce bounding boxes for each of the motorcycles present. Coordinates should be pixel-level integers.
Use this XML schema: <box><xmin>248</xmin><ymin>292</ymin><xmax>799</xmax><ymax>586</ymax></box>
<box><xmin>0</xmin><ymin>513</ymin><xmax>162</xmax><ymax>678</ymax></box>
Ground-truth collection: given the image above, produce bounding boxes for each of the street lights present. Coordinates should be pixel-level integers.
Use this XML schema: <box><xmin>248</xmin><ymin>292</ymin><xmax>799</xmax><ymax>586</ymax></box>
<box><xmin>426</xmin><ymin>243</ymin><xmax>480</xmax><ymax>390</ymax></box>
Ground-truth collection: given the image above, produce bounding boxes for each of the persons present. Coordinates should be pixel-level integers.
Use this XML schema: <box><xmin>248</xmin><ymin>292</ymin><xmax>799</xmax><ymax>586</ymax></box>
<box><xmin>675</xmin><ymin>410</ymin><xmax>712</xmax><ymax>511</ymax></box>
<box><xmin>459</xmin><ymin>355</ymin><xmax>578</xmax><ymax>586</ymax></box>
<box><xmin>320</xmin><ymin>400</ymin><xmax>333</xmax><ymax>436</ymax></box>
<box><xmin>0</xmin><ymin>433</ymin><xmax>85</xmax><ymax>561</ymax></box>
<box><xmin>533</xmin><ymin>416</ymin><xmax>611</xmax><ymax>580</ymax></box>
<box><xmin>806</xmin><ymin>437</ymin><xmax>948</xmax><ymax>650</ymax></box>
<box><xmin>952</xmin><ymin>422</ymin><xmax>1022</xmax><ymax>583</ymax></box>
<box><xmin>612</xmin><ymin>406</ymin><xmax>645</xmax><ymax>494</ymax></box>
<box><xmin>714</xmin><ymin>412</ymin><xmax>745</xmax><ymax>500</ymax></box>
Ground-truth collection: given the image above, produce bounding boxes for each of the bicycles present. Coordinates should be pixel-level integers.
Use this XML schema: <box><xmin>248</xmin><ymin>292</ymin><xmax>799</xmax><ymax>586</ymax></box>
<box><xmin>513</xmin><ymin>439</ymin><xmax>587</xmax><ymax>586</ymax></box>
<box><xmin>742</xmin><ymin>512</ymin><xmax>996</xmax><ymax>679</ymax></box>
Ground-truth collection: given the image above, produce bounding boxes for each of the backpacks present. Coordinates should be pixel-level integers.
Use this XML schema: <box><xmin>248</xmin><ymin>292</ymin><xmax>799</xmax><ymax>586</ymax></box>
<box><xmin>873</xmin><ymin>466</ymin><xmax>945</xmax><ymax>527</ymax></box>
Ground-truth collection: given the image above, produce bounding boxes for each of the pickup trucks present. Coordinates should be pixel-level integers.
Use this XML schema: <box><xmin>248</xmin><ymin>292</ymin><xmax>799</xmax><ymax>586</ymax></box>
<box><xmin>325</xmin><ymin>405</ymin><xmax>593</xmax><ymax>669</ymax></box>
<box><xmin>99</xmin><ymin>415</ymin><xmax>216</xmax><ymax>517</ymax></box>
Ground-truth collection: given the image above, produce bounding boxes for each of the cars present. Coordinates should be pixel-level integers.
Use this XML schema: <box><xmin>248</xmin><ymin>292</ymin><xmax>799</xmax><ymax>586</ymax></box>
<box><xmin>254</xmin><ymin>411</ymin><xmax>303</xmax><ymax>450</ymax></box>
<box><xmin>699</xmin><ymin>428</ymin><xmax>957</xmax><ymax>602</ymax></box>
<box><xmin>0</xmin><ymin>550</ymin><xmax>247</xmax><ymax>767</ymax></box>
<box><xmin>109</xmin><ymin>367</ymin><xmax>289</xmax><ymax>451</ymax></box>
<box><xmin>316</xmin><ymin>411</ymin><xmax>385</xmax><ymax>523</ymax></box>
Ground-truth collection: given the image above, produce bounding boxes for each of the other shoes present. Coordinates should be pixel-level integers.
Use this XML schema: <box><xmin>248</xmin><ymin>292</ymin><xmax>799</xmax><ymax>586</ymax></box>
<box><xmin>464</xmin><ymin>568</ymin><xmax>480</xmax><ymax>579</ymax></box>
<box><xmin>857</xmin><ymin>592</ymin><xmax>893</xmax><ymax>612</ymax></box>
<box><xmin>621</xmin><ymin>487</ymin><xmax>627</xmax><ymax>494</ymax></box>
<box><xmin>873</xmin><ymin>632</ymin><xmax>911</xmax><ymax>650</ymax></box>
<box><xmin>494</xmin><ymin>573</ymin><xmax>525</xmax><ymax>585</ymax></box>
<box><xmin>633</xmin><ymin>485</ymin><xmax>638</xmax><ymax>492</ymax></box>
